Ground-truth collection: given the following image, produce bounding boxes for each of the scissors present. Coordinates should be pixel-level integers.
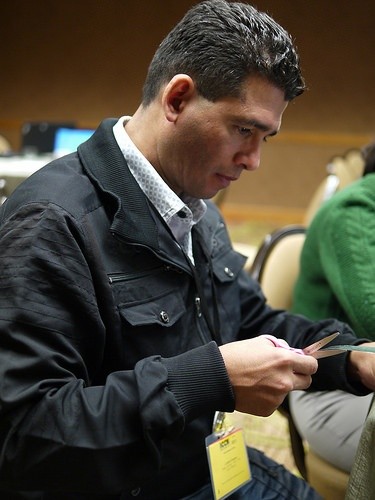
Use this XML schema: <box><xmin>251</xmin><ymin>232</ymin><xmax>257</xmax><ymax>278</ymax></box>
<box><xmin>267</xmin><ymin>332</ymin><xmax>347</xmax><ymax>359</ymax></box>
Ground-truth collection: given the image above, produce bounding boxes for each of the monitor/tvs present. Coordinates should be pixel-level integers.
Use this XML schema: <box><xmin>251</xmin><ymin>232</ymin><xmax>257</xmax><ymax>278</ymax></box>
<box><xmin>54</xmin><ymin>128</ymin><xmax>97</xmax><ymax>159</ymax></box>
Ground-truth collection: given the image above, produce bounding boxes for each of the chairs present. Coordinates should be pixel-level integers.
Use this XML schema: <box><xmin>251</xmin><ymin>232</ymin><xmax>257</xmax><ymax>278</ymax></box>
<box><xmin>249</xmin><ymin>226</ymin><xmax>352</xmax><ymax>499</ymax></box>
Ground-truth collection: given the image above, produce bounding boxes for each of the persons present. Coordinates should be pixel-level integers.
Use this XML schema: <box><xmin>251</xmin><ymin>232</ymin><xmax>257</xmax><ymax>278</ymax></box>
<box><xmin>288</xmin><ymin>146</ymin><xmax>375</xmax><ymax>474</ymax></box>
<box><xmin>0</xmin><ymin>1</ymin><xmax>375</xmax><ymax>500</ymax></box>
